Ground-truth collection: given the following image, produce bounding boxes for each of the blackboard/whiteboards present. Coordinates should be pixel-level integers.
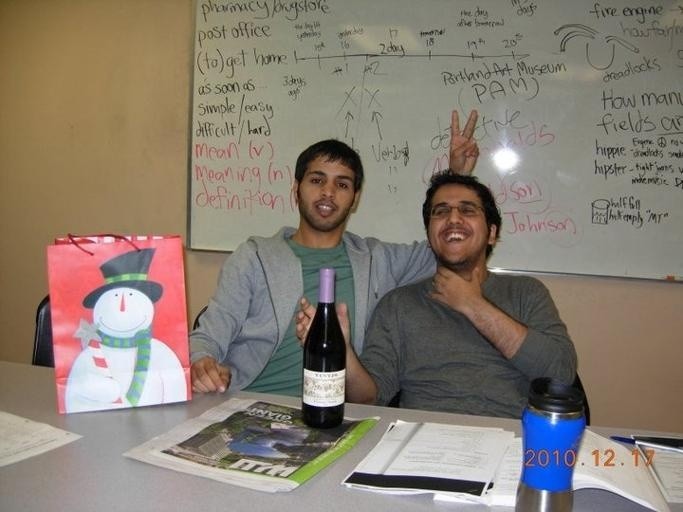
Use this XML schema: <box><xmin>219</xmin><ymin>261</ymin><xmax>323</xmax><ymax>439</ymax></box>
<box><xmin>187</xmin><ymin>1</ymin><xmax>681</xmax><ymax>283</ymax></box>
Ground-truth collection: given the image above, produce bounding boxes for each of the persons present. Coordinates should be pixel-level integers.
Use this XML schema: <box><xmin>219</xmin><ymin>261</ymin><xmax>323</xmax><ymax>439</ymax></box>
<box><xmin>293</xmin><ymin>167</ymin><xmax>579</xmax><ymax>421</ymax></box>
<box><xmin>188</xmin><ymin>109</ymin><xmax>480</xmax><ymax>394</ymax></box>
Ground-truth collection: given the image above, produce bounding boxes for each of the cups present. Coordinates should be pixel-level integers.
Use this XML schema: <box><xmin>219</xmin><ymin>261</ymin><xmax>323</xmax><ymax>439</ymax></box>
<box><xmin>513</xmin><ymin>375</ymin><xmax>588</xmax><ymax>512</ymax></box>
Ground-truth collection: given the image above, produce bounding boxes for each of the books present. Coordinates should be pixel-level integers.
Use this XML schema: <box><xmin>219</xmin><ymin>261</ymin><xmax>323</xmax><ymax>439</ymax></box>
<box><xmin>343</xmin><ymin>417</ymin><xmax>683</xmax><ymax>511</ymax></box>
<box><xmin>157</xmin><ymin>400</ymin><xmax>383</xmax><ymax>494</ymax></box>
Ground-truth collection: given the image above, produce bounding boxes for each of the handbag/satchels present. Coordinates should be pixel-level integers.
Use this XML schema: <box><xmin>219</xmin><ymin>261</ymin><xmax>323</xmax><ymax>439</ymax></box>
<box><xmin>44</xmin><ymin>232</ymin><xmax>192</xmax><ymax>415</ymax></box>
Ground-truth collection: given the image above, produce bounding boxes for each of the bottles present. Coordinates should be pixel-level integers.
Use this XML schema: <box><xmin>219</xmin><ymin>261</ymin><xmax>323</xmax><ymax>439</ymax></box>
<box><xmin>300</xmin><ymin>268</ymin><xmax>347</xmax><ymax>430</ymax></box>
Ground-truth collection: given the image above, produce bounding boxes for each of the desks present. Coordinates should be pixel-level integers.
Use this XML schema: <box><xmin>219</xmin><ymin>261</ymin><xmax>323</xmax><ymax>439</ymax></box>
<box><xmin>1</xmin><ymin>357</ymin><xmax>683</xmax><ymax>512</ymax></box>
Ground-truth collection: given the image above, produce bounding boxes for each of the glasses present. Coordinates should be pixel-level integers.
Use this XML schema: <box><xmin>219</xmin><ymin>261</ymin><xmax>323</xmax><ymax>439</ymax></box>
<box><xmin>424</xmin><ymin>204</ymin><xmax>485</xmax><ymax>218</ymax></box>
